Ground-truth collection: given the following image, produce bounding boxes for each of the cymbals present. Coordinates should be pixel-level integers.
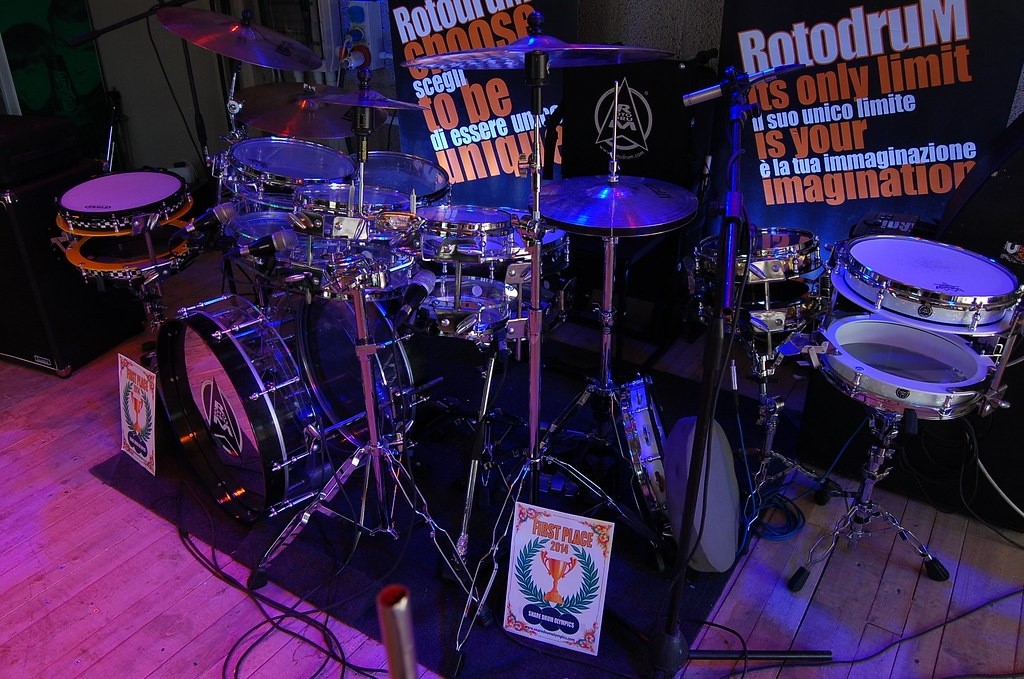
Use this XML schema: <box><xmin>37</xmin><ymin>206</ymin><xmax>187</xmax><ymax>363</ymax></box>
<box><xmin>307</xmin><ymin>87</ymin><xmax>432</xmax><ymax>115</ymax></box>
<box><xmin>531</xmin><ymin>172</ymin><xmax>702</xmax><ymax>239</ymax></box>
<box><xmin>234</xmin><ymin>85</ymin><xmax>388</xmax><ymax>143</ymax></box>
<box><xmin>397</xmin><ymin>33</ymin><xmax>679</xmax><ymax>75</ymax></box>
<box><xmin>150</xmin><ymin>3</ymin><xmax>324</xmax><ymax>73</ymax></box>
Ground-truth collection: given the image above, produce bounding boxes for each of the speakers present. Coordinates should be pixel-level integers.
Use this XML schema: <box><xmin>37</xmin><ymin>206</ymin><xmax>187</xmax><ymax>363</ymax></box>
<box><xmin>1</xmin><ymin>0</ymin><xmax>150</xmax><ymax>379</ymax></box>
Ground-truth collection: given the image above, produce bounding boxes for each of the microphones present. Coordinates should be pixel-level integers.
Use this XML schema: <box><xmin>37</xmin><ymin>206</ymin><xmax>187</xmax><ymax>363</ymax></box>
<box><xmin>343</xmin><ymin>106</ymin><xmax>355</xmax><ymax>120</ymax></box>
<box><xmin>393</xmin><ymin>269</ymin><xmax>436</xmax><ymax>328</ymax></box>
<box><xmin>224</xmin><ymin>228</ymin><xmax>299</xmax><ymax>260</ymax></box>
<box><xmin>700</xmin><ymin>154</ymin><xmax>712</xmax><ymax>226</ymax></box>
<box><xmin>682</xmin><ymin>62</ymin><xmax>805</xmax><ymax>107</ymax></box>
<box><xmin>544</xmin><ymin>99</ymin><xmax>573</xmax><ymax>128</ymax></box>
<box><xmin>177</xmin><ymin>203</ymin><xmax>238</xmax><ymax>237</ymax></box>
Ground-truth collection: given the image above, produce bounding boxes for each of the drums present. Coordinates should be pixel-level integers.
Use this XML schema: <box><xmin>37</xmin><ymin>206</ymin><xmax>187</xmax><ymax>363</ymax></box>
<box><xmin>810</xmin><ymin>232</ymin><xmax>1018</xmax><ymax>429</ymax></box>
<box><xmin>47</xmin><ymin>165</ymin><xmax>199</xmax><ymax>288</ymax></box>
<box><xmin>619</xmin><ymin>372</ymin><xmax>673</xmax><ymax>537</ymax></box>
<box><xmin>208</xmin><ymin>134</ymin><xmax>358</xmax><ymax>276</ymax></box>
<box><xmin>287</xmin><ymin>184</ymin><xmax>418</xmax><ymax>299</ymax></box>
<box><xmin>152</xmin><ymin>286</ymin><xmax>421</xmax><ymax>524</ymax></box>
<box><xmin>348</xmin><ymin>149</ymin><xmax>451</xmax><ymax>215</ymax></box>
<box><xmin>694</xmin><ymin>226</ymin><xmax>826</xmax><ymax>337</ymax></box>
<box><xmin>415</xmin><ymin>201</ymin><xmax>522</xmax><ymax>337</ymax></box>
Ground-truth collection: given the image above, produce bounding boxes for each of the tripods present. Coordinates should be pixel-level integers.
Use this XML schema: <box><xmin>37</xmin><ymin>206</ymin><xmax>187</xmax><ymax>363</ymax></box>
<box><xmin>250</xmin><ymin>236</ymin><xmax>952</xmax><ymax>679</ymax></box>
